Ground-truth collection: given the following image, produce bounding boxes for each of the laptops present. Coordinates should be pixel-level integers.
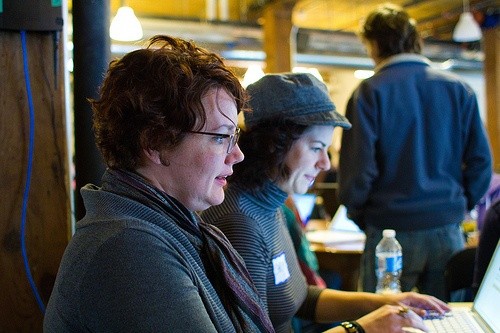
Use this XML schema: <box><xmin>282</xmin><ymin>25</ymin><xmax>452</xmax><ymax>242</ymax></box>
<box><xmin>402</xmin><ymin>238</ymin><xmax>500</xmax><ymax>333</ymax></box>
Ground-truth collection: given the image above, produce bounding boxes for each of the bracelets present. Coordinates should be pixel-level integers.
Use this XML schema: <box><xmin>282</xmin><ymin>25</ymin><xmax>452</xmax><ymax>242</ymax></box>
<box><xmin>341</xmin><ymin>321</ymin><xmax>368</xmax><ymax>333</ymax></box>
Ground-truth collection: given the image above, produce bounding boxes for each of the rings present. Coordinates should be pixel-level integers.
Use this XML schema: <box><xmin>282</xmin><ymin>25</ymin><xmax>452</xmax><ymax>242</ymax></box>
<box><xmin>400</xmin><ymin>306</ymin><xmax>409</xmax><ymax>315</ymax></box>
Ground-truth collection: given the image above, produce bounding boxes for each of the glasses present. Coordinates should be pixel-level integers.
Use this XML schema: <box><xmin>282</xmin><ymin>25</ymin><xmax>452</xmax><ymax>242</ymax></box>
<box><xmin>183</xmin><ymin>128</ymin><xmax>240</xmax><ymax>154</ymax></box>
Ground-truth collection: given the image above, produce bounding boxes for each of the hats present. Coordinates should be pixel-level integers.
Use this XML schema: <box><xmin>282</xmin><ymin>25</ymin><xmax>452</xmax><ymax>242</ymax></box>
<box><xmin>243</xmin><ymin>73</ymin><xmax>352</xmax><ymax>128</ymax></box>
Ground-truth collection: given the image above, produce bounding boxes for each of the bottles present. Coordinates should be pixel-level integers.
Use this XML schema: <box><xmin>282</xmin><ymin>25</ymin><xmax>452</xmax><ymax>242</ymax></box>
<box><xmin>375</xmin><ymin>229</ymin><xmax>403</xmax><ymax>294</ymax></box>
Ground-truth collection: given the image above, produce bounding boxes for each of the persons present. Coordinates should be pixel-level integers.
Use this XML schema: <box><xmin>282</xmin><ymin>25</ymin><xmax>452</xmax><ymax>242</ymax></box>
<box><xmin>200</xmin><ymin>75</ymin><xmax>448</xmax><ymax>333</ymax></box>
<box><xmin>339</xmin><ymin>6</ymin><xmax>493</xmax><ymax>301</ymax></box>
<box><xmin>41</xmin><ymin>34</ymin><xmax>276</xmax><ymax>333</ymax></box>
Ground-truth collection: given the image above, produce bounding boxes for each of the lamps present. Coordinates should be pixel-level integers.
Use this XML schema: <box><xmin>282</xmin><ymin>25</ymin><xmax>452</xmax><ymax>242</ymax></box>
<box><xmin>110</xmin><ymin>0</ymin><xmax>143</xmax><ymax>41</ymax></box>
<box><xmin>452</xmin><ymin>0</ymin><xmax>483</xmax><ymax>42</ymax></box>
